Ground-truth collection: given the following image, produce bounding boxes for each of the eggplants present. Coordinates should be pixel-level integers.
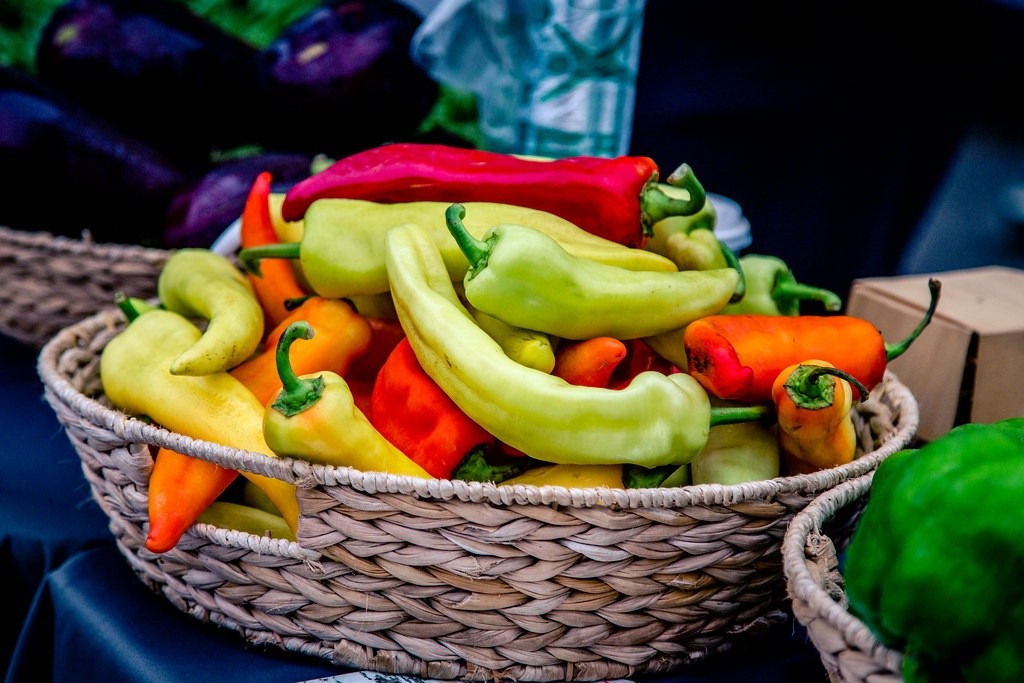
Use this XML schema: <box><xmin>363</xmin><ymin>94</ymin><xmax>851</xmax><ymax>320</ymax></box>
<box><xmin>0</xmin><ymin>0</ymin><xmax>444</xmax><ymax>250</ymax></box>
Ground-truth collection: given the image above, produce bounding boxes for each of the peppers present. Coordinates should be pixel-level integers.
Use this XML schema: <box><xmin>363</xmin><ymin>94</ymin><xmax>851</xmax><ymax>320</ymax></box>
<box><xmin>846</xmin><ymin>417</ymin><xmax>1024</xmax><ymax>683</ymax></box>
<box><xmin>99</xmin><ymin>144</ymin><xmax>942</xmax><ymax>552</ymax></box>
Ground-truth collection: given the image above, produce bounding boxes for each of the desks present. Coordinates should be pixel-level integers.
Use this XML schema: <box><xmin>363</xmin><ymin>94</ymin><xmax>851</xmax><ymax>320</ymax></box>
<box><xmin>1</xmin><ymin>334</ymin><xmax>836</xmax><ymax>683</ymax></box>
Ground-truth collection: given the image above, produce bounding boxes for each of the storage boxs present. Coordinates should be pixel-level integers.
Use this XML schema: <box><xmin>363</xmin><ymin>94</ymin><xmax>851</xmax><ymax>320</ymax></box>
<box><xmin>845</xmin><ymin>265</ymin><xmax>1024</xmax><ymax>443</ymax></box>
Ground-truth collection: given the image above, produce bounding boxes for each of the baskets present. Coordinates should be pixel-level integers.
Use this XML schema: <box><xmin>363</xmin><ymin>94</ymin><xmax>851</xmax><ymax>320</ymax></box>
<box><xmin>36</xmin><ymin>298</ymin><xmax>920</xmax><ymax>682</ymax></box>
<box><xmin>780</xmin><ymin>472</ymin><xmax>919</xmax><ymax>683</ymax></box>
<box><xmin>0</xmin><ymin>228</ymin><xmax>180</xmax><ymax>346</ymax></box>
